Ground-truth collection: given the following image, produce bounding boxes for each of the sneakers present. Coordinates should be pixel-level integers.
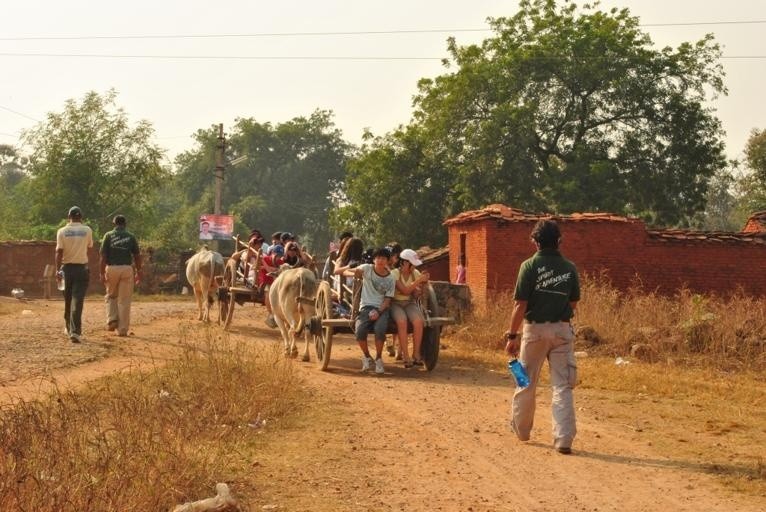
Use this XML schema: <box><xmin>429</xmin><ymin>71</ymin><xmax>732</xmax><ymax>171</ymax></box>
<box><xmin>374</xmin><ymin>358</ymin><xmax>384</xmax><ymax>374</ymax></box>
<box><xmin>361</xmin><ymin>357</ymin><xmax>375</xmax><ymax>371</ymax></box>
<box><xmin>511</xmin><ymin>419</ymin><xmax>530</xmax><ymax>440</ymax></box>
<box><xmin>64</xmin><ymin>328</ymin><xmax>80</xmax><ymax>343</ymax></box>
<box><xmin>108</xmin><ymin>320</ymin><xmax>127</xmax><ymax>336</ymax></box>
<box><xmin>554</xmin><ymin>441</ymin><xmax>572</xmax><ymax>454</ymax></box>
<box><xmin>404</xmin><ymin>356</ymin><xmax>424</xmax><ymax>369</ymax></box>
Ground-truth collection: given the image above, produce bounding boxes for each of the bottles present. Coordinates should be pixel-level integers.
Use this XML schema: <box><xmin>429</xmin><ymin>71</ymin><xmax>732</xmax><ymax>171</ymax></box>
<box><xmin>508</xmin><ymin>357</ymin><xmax>530</xmax><ymax>389</ymax></box>
<box><xmin>57</xmin><ymin>270</ymin><xmax>65</xmax><ymax>290</ymax></box>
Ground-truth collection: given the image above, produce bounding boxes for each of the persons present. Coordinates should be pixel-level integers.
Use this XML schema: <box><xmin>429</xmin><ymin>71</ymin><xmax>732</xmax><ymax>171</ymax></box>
<box><xmin>98</xmin><ymin>215</ymin><xmax>142</xmax><ymax>336</ymax></box>
<box><xmin>323</xmin><ymin>232</ymin><xmax>430</xmax><ymax>374</ymax></box>
<box><xmin>232</xmin><ymin>230</ymin><xmax>303</xmax><ymax>327</ymax></box>
<box><xmin>506</xmin><ymin>217</ymin><xmax>580</xmax><ymax>454</ymax></box>
<box><xmin>56</xmin><ymin>206</ymin><xmax>93</xmax><ymax>343</ymax></box>
<box><xmin>200</xmin><ymin>222</ymin><xmax>213</xmax><ymax>240</ymax></box>
<box><xmin>455</xmin><ymin>254</ymin><xmax>466</xmax><ymax>284</ymax></box>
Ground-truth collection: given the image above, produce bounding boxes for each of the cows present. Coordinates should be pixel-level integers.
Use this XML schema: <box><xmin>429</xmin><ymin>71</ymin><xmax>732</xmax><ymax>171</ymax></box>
<box><xmin>185</xmin><ymin>250</ymin><xmax>228</xmax><ymax>326</ymax></box>
<box><xmin>267</xmin><ymin>267</ymin><xmax>318</xmax><ymax>361</ymax></box>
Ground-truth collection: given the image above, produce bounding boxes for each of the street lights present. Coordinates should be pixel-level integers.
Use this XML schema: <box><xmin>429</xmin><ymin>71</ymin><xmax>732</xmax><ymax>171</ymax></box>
<box><xmin>211</xmin><ymin>153</ymin><xmax>248</xmax><ymax>254</ymax></box>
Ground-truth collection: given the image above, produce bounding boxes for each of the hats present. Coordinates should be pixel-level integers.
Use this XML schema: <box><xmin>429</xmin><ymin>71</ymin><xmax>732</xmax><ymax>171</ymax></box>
<box><xmin>251</xmin><ymin>231</ymin><xmax>299</xmax><ymax>254</ymax></box>
<box><xmin>69</xmin><ymin>206</ymin><xmax>82</xmax><ymax>215</ymax></box>
<box><xmin>400</xmin><ymin>248</ymin><xmax>424</xmax><ymax>267</ymax></box>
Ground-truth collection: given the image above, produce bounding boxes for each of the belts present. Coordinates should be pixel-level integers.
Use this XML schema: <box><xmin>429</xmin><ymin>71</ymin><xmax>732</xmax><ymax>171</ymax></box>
<box><xmin>106</xmin><ymin>262</ymin><xmax>132</xmax><ymax>267</ymax></box>
<box><xmin>522</xmin><ymin>317</ymin><xmax>573</xmax><ymax>326</ymax></box>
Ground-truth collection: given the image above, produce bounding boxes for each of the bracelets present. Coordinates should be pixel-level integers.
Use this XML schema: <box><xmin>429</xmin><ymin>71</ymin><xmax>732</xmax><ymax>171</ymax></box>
<box><xmin>506</xmin><ymin>333</ymin><xmax>516</xmax><ymax>339</ymax></box>
